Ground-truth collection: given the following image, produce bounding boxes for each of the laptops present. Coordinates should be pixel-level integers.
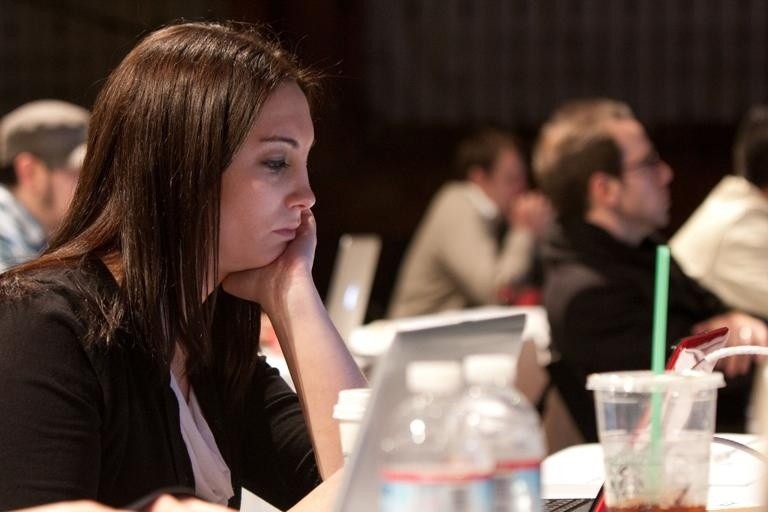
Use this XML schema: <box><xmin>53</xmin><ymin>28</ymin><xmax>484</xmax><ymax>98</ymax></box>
<box><xmin>323</xmin><ymin>232</ymin><xmax>382</xmax><ymax>338</ymax></box>
<box><xmin>332</xmin><ymin>312</ymin><xmax>529</xmax><ymax>512</ymax></box>
<box><xmin>531</xmin><ymin>326</ymin><xmax>730</xmax><ymax>512</ymax></box>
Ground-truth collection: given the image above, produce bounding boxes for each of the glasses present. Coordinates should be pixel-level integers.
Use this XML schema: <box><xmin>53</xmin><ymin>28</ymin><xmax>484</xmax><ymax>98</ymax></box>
<box><xmin>625</xmin><ymin>147</ymin><xmax>665</xmax><ymax>177</ymax></box>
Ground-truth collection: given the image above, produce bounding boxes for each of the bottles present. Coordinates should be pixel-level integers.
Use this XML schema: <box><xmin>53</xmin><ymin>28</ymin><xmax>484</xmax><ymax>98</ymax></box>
<box><xmin>378</xmin><ymin>361</ymin><xmax>456</xmax><ymax>512</ymax></box>
<box><xmin>448</xmin><ymin>350</ymin><xmax>545</xmax><ymax>512</ymax></box>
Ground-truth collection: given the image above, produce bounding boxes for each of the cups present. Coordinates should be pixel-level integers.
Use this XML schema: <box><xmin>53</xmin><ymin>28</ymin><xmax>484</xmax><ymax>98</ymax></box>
<box><xmin>333</xmin><ymin>389</ymin><xmax>371</xmax><ymax>464</ymax></box>
<box><xmin>589</xmin><ymin>371</ymin><xmax>724</xmax><ymax>510</ymax></box>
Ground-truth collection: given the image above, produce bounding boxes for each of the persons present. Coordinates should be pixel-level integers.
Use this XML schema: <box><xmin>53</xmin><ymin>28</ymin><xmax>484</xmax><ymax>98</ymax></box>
<box><xmin>0</xmin><ymin>97</ymin><xmax>93</xmax><ymax>272</ymax></box>
<box><xmin>0</xmin><ymin>18</ymin><xmax>369</xmax><ymax>512</ymax></box>
<box><xmin>666</xmin><ymin>100</ymin><xmax>767</xmax><ymax>324</ymax></box>
<box><xmin>531</xmin><ymin>96</ymin><xmax>767</xmax><ymax>444</ymax></box>
<box><xmin>384</xmin><ymin>124</ymin><xmax>556</xmax><ymax>321</ymax></box>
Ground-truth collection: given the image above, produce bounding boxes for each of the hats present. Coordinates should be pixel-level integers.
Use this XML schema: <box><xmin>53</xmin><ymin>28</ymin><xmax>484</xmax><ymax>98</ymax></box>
<box><xmin>0</xmin><ymin>97</ymin><xmax>92</xmax><ymax>170</ymax></box>
